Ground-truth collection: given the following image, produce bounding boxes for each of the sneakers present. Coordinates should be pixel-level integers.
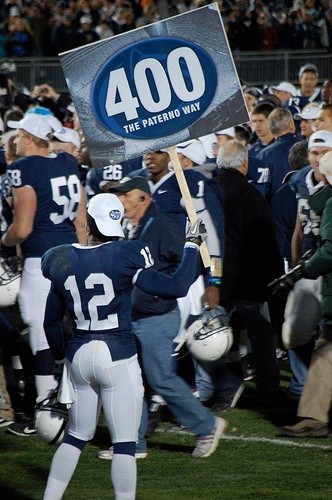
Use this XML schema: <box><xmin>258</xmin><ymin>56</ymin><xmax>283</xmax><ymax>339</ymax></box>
<box><xmin>6</xmin><ymin>420</ymin><xmax>40</xmax><ymax>437</ymax></box>
<box><xmin>98</xmin><ymin>444</ymin><xmax>149</xmax><ymax>460</ymax></box>
<box><xmin>191</xmin><ymin>416</ymin><xmax>229</xmax><ymax>459</ymax></box>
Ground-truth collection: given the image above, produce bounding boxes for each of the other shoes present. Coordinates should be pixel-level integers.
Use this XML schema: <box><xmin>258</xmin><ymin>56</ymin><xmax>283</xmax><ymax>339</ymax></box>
<box><xmin>150</xmin><ymin>385</ymin><xmax>200</xmax><ymax>405</ymax></box>
<box><xmin>210</xmin><ymin>382</ymin><xmax>245</xmax><ymax>413</ymax></box>
<box><xmin>243</xmin><ymin>367</ymin><xmax>256</xmax><ymax>381</ymax></box>
<box><xmin>280</xmin><ymin>419</ymin><xmax>329</xmax><ymax>439</ymax></box>
<box><xmin>0</xmin><ymin>415</ymin><xmax>15</xmax><ymax>428</ymax></box>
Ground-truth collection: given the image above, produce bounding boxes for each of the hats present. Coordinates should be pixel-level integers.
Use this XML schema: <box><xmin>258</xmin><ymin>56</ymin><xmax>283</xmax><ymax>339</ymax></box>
<box><xmin>160</xmin><ymin>138</ymin><xmax>206</xmax><ymax>166</ymax></box>
<box><xmin>293</xmin><ymin>102</ymin><xmax>321</xmax><ymax>120</ymax></box>
<box><xmin>307</xmin><ymin>129</ymin><xmax>332</xmax><ymax>149</ymax></box>
<box><xmin>7</xmin><ymin>113</ymin><xmax>81</xmax><ymax>150</ymax></box>
<box><xmin>198</xmin><ymin>133</ymin><xmax>217</xmax><ymax>159</ymax></box>
<box><xmin>10</xmin><ymin>5</ymin><xmax>20</xmax><ymax>17</ymax></box>
<box><xmin>80</xmin><ymin>15</ymin><xmax>92</xmax><ymax>25</ymax></box>
<box><xmin>299</xmin><ymin>63</ymin><xmax>318</xmax><ymax>78</ymax></box>
<box><xmin>318</xmin><ymin>151</ymin><xmax>332</xmax><ymax>186</ymax></box>
<box><xmin>243</xmin><ymin>87</ymin><xmax>263</xmax><ymax>100</ymax></box>
<box><xmin>268</xmin><ymin>81</ymin><xmax>297</xmax><ymax>96</ymax></box>
<box><xmin>108</xmin><ymin>175</ymin><xmax>152</xmax><ymax>195</ymax></box>
<box><xmin>214</xmin><ymin>126</ymin><xmax>235</xmax><ymax>138</ymax></box>
<box><xmin>87</xmin><ymin>193</ymin><xmax>126</xmax><ymax>238</ymax></box>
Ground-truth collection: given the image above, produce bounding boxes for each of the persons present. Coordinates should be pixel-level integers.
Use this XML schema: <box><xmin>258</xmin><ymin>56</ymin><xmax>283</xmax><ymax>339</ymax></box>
<box><xmin>0</xmin><ymin>0</ymin><xmax>332</xmax><ymax>452</ymax></box>
<box><xmin>41</xmin><ymin>193</ymin><xmax>210</xmax><ymax>500</ymax></box>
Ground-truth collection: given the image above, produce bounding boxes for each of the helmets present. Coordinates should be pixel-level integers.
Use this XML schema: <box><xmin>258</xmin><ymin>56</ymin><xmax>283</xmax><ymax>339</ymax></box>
<box><xmin>174</xmin><ymin>318</ymin><xmax>234</xmax><ymax>362</ymax></box>
<box><xmin>33</xmin><ymin>406</ymin><xmax>69</xmax><ymax>446</ymax></box>
